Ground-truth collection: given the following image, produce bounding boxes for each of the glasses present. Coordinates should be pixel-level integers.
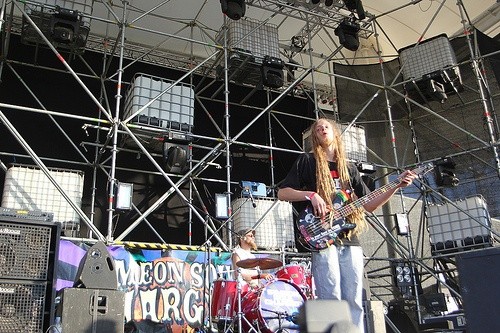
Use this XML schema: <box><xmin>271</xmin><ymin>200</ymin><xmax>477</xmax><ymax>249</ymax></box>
<box><xmin>246</xmin><ymin>235</ymin><xmax>252</xmax><ymax>237</ymax></box>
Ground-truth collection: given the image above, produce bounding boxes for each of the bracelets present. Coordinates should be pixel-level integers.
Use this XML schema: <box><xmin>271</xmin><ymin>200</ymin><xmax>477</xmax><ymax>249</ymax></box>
<box><xmin>257</xmin><ymin>273</ymin><xmax>261</xmax><ymax>279</ymax></box>
<box><xmin>304</xmin><ymin>192</ymin><xmax>316</xmax><ymax>200</ymax></box>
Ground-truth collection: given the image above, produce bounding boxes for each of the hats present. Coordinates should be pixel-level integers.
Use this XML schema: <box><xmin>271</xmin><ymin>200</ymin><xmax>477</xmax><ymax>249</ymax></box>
<box><xmin>237</xmin><ymin>230</ymin><xmax>255</xmax><ymax>241</ymax></box>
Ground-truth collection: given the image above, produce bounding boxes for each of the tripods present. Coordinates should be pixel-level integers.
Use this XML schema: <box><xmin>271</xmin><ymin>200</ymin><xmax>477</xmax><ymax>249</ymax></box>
<box><xmin>223</xmin><ymin>267</ymin><xmax>259</xmax><ymax>333</ymax></box>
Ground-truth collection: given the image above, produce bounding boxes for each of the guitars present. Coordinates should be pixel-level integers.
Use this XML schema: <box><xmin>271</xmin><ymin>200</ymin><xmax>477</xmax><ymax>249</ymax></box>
<box><xmin>297</xmin><ymin>161</ymin><xmax>435</xmax><ymax>249</ymax></box>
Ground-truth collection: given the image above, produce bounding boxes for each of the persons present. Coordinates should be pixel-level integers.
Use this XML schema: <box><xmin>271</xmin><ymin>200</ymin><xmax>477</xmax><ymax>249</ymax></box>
<box><xmin>275</xmin><ymin>118</ymin><xmax>418</xmax><ymax>333</ymax></box>
<box><xmin>231</xmin><ymin>228</ymin><xmax>274</xmax><ymax>291</ymax></box>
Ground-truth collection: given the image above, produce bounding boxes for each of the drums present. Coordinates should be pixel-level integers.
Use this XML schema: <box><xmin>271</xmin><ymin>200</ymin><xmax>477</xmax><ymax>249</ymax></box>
<box><xmin>276</xmin><ymin>264</ymin><xmax>309</xmax><ymax>293</ymax></box>
<box><xmin>235</xmin><ymin>278</ymin><xmax>308</xmax><ymax>333</ymax></box>
<box><xmin>211</xmin><ymin>280</ymin><xmax>238</xmax><ymax>322</ymax></box>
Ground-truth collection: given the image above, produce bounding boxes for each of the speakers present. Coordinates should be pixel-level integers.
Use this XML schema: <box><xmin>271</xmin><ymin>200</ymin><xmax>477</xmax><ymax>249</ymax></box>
<box><xmin>59</xmin><ymin>287</ymin><xmax>125</xmax><ymax>333</ymax></box>
<box><xmin>74</xmin><ymin>240</ymin><xmax>118</xmax><ymax>289</ymax></box>
<box><xmin>0</xmin><ymin>278</ymin><xmax>47</xmax><ymax>333</ymax></box>
<box><xmin>1</xmin><ymin>223</ymin><xmax>52</xmax><ymax>280</ymax></box>
<box><xmin>298</xmin><ymin>299</ymin><xmax>352</xmax><ymax>333</ymax></box>
<box><xmin>454</xmin><ymin>244</ymin><xmax>500</xmax><ymax>333</ymax></box>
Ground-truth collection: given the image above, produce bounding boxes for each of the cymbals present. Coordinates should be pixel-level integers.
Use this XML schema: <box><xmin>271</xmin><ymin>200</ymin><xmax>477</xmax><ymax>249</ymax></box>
<box><xmin>236</xmin><ymin>258</ymin><xmax>283</xmax><ymax>270</ymax></box>
